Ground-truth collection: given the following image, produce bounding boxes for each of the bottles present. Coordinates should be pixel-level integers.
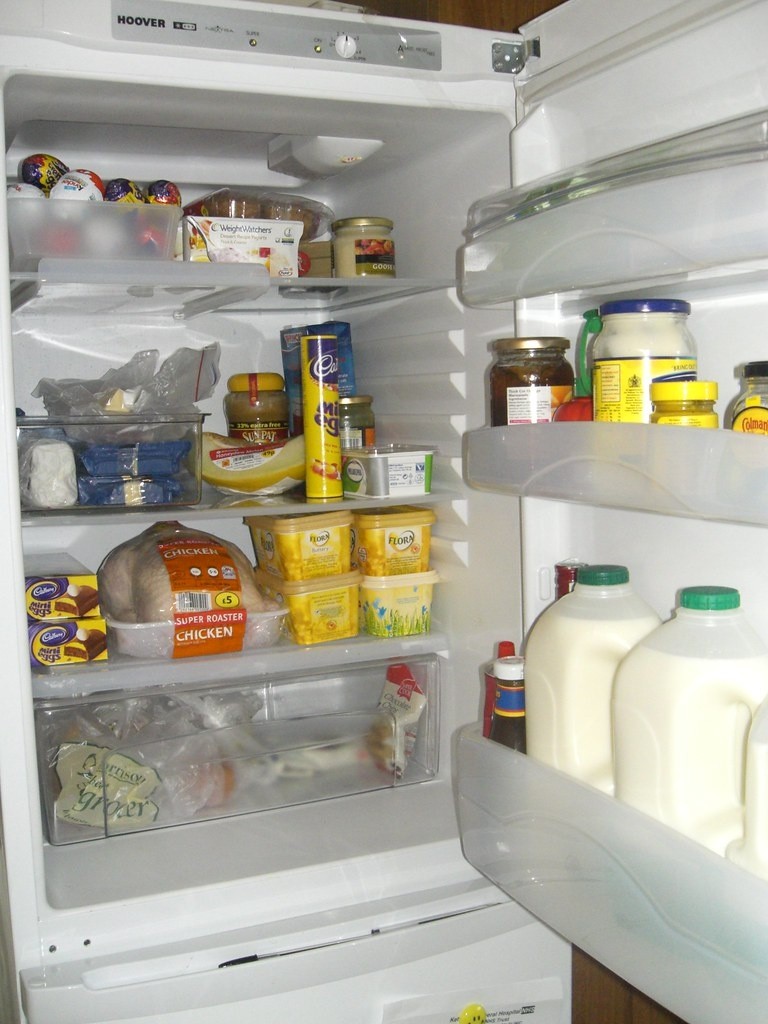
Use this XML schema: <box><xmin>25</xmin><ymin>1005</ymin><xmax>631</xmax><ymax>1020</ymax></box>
<box><xmin>484</xmin><ymin>641</ymin><xmax>515</xmax><ymax>737</ymax></box>
<box><xmin>523</xmin><ymin>564</ymin><xmax>664</xmax><ymax>796</ymax></box>
<box><xmin>611</xmin><ymin>586</ymin><xmax>768</xmax><ymax>881</ymax></box>
<box><xmin>732</xmin><ymin>361</ymin><xmax>768</xmax><ymax>433</ymax></box>
<box><xmin>489</xmin><ymin>656</ymin><xmax>527</xmax><ymax>754</ymax></box>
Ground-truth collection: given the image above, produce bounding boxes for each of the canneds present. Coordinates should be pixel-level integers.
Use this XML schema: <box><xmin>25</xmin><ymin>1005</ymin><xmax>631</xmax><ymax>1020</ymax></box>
<box><xmin>221</xmin><ymin>372</ymin><xmax>289</xmax><ymax>441</ymax></box>
<box><xmin>487</xmin><ymin>296</ymin><xmax>718</xmax><ymax>430</ymax></box>
<box><xmin>330</xmin><ymin>216</ymin><xmax>396</xmax><ymax>279</ymax></box>
<box><xmin>337</xmin><ymin>394</ymin><xmax>375</xmax><ymax>449</ymax></box>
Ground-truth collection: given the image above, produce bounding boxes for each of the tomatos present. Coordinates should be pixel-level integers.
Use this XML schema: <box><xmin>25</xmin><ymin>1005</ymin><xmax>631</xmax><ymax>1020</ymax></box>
<box><xmin>297</xmin><ymin>252</ymin><xmax>310</xmax><ymax>276</ymax></box>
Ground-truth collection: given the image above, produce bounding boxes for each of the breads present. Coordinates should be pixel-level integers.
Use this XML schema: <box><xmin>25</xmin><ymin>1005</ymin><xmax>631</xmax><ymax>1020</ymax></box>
<box><xmin>180</xmin><ymin>189</ymin><xmax>322</xmax><ymax>240</ymax></box>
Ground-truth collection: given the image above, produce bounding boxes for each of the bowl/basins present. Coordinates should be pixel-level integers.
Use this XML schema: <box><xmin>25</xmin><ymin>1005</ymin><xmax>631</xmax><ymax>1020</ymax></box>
<box><xmin>99</xmin><ymin>587</ymin><xmax>291</xmax><ymax>659</ymax></box>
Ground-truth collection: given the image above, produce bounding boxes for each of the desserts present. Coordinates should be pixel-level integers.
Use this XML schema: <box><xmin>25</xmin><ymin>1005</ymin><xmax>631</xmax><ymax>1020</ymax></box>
<box><xmin>54</xmin><ymin>585</ymin><xmax>107</xmax><ymax>661</ymax></box>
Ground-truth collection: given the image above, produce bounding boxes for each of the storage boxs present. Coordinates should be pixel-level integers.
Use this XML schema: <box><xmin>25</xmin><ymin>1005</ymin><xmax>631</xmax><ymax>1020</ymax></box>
<box><xmin>16</xmin><ymin>413</ymin><xmax>212</xmax><ymax>512</ymax></box>
<box><xmin>7</xmin><ymin>185</ymin><xmax>335</xmax><ymax>278</ymax></box>
<box><xmin>281</xmin><ymin>321</ymin><xmax>355</xmax><ymax>438</ymax></box>
<box><xmin>25</xmin><ymin>577</ymin><xmax>108</xmax><ymax>667</ymax></box>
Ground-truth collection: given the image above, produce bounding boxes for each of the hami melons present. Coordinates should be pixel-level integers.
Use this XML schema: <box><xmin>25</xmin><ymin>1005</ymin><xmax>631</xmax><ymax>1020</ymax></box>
<box><xmin>183</xmin><ymin>432</ymin><xmax>306</xmax><ymax>491</ymax></box>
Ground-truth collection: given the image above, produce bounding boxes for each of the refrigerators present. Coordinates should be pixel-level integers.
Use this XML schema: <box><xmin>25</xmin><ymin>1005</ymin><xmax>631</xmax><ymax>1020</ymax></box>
<box><xmin>0</xmin><ymin>0</ymin><xmax>768</xmax><ymax>1023</ymax></box>
